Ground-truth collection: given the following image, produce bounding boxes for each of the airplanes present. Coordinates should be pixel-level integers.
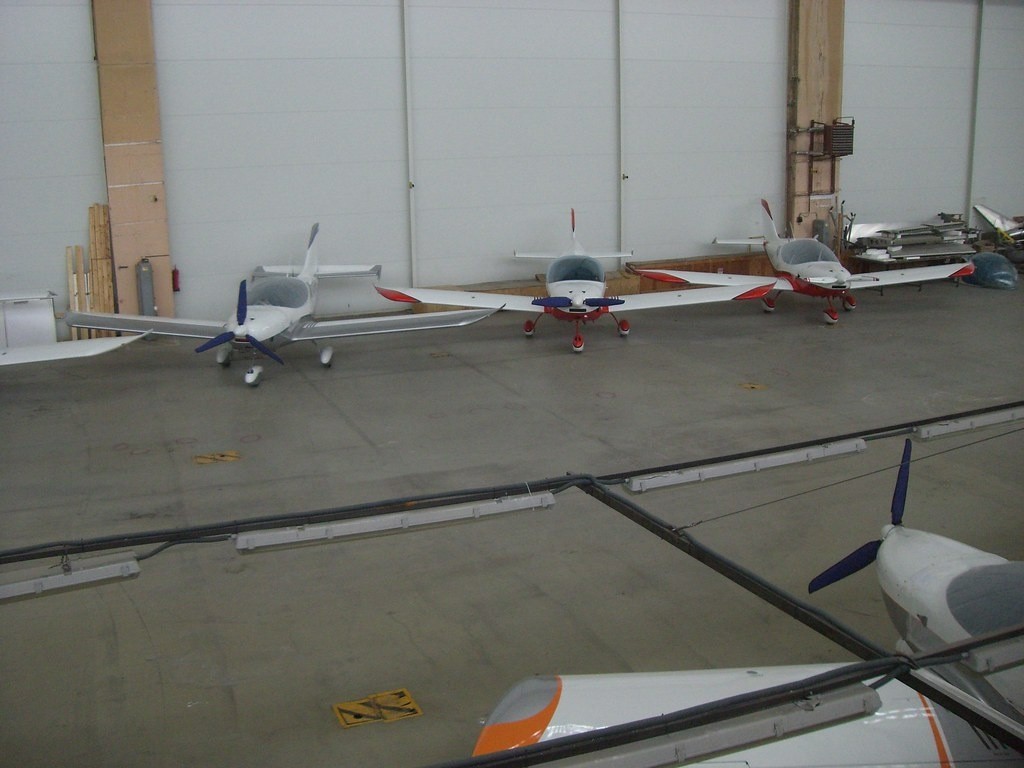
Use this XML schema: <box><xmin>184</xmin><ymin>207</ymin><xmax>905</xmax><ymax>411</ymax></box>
<box><xmin>626</xmin><ymin>197</ymin><xmax>976</xmax><ymax>324</ymax></box>
<box><xmin>63</xmin><ymin>221</ymin><xmax>508</xmax><ymax>386</ymax></box>
<box><xmin>368</xmin><ymin>206</ymin><xmax>778</xmax><ymax>353</ymax></box>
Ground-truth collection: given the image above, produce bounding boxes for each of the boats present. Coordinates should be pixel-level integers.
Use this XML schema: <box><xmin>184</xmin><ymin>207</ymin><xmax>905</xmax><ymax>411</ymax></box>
<box><xmin>463</xmin><ymin>436</ymin><xmax>1023</xmax><ymax>767</ymax></box>
